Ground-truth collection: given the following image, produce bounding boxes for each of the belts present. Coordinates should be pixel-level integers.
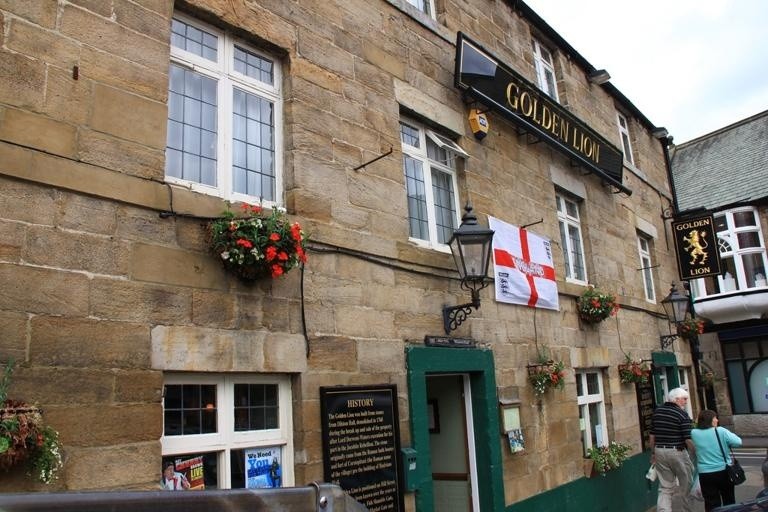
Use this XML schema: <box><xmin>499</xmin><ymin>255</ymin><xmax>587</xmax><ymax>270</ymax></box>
<box><xmin>655</xmin><ymin>444</ymin><xmax>686</xmax><ymax>449</ymax></box>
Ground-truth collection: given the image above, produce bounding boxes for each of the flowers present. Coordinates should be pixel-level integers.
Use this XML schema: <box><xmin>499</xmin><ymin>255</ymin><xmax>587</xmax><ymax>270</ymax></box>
<box><xmin>585</xmin><ymin>440</ymin><xmax>639</xmax><ymax>478</ymax></box>
<box><xmin>203</xmin><ymin>194</ymin><xmax>315</xmax><ymax>283</ymax></box>
<box><xmin>527</xmin><ymin>343</ymin><xmax>568</xmax><ymax>401</ymax></box>
<box><xmin>0</xmin><ymin>354</ymin><xmax>68</xmax><ymax>487</ymax></box>
<box><xmin>618</xmin><ymin>350</ymin><xmax>653</xmax><ymax>387</ymax></box>
<box><xmin>577</xmin><ymin>285</ymin><xmax>622</xmax><ymax>326</ymax></box>
<box><xmin>673</xmin><ymin>315</ymin><xmax>706</xmax><ymax>338</ymax></box>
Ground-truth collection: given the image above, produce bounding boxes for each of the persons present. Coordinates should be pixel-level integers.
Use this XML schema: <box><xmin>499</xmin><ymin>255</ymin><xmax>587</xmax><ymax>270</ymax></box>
<box><xmin>649</xmin><ymin>387</ymin><xmax>743</xmax><ymax>512</ymax></box>
<box><xmin>163</xmin><ymin>461</ymin><xmax>191</xmax><ymax>492</ymax></box>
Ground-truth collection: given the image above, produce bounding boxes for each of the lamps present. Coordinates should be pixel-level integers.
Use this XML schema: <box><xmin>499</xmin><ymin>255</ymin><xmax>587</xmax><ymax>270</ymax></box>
<box><xmin>440</xmin><ymin>202</ymin><xmax>496</xmax><ymax>334</ymax></box>
<box><xmin>660</xmin><ymin>281</ymin><xmax>692</xmax><ymax>351</ymax></box>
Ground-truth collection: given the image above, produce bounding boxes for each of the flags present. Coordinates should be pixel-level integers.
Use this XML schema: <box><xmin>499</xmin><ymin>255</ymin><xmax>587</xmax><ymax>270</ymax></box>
<box><xmin>487</xmin><ymin>214</ymin><xmax>559</xmax><ymax>312</ymax></box>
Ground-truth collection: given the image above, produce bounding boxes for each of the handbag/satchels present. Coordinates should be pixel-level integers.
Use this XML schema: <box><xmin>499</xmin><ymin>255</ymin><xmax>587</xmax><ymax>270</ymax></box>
<box><xmin>725</xmin><ymin>458</ymin><xmax>746</xmax><ymax>486</ymax></box>
